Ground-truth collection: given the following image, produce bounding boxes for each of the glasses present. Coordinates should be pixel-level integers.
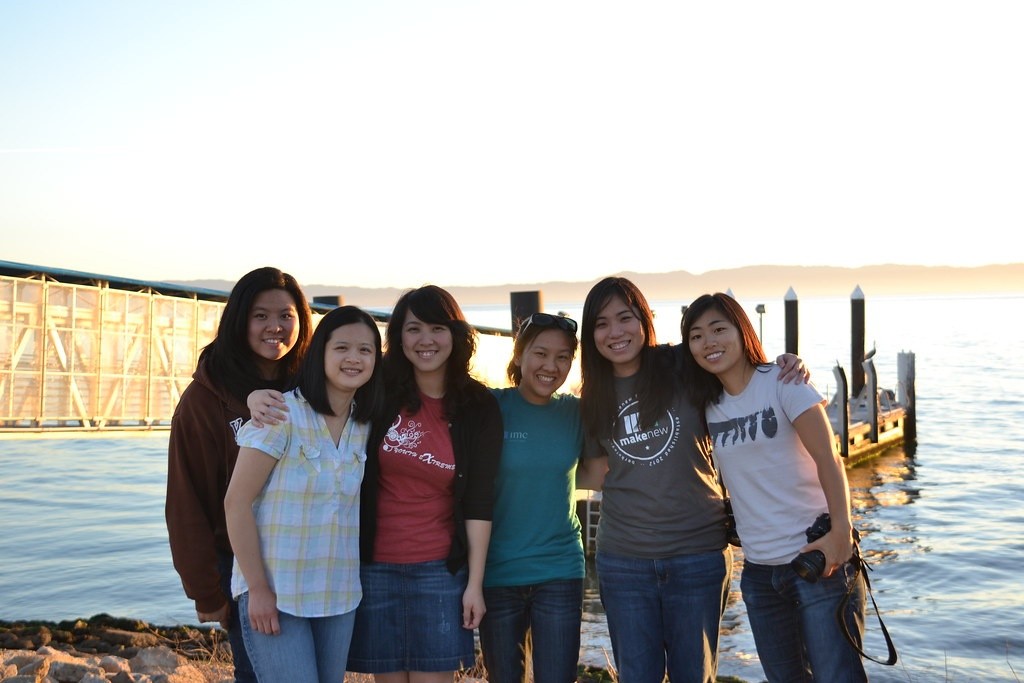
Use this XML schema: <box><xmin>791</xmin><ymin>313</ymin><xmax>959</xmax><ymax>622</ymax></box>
<box><xmin>522</xmin><ymin>312</ymin><xmax>578</xmax><ymax>338</ymax></box>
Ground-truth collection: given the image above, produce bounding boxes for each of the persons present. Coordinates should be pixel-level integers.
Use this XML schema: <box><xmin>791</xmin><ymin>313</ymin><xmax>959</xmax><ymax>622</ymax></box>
<box><xmin>223</xmin><ymin>305</ymin><xmax>386</xmax><ymax>683</ymax></box>
<box><xmin>680</xmin><ymin>293</ymin><xmax>867</xmax><ymax>683</ymax></box>
<box><xmin>245</xmin><ymin>285</ymin><xmax>503</xmax><ymax>683</ymax></box>
<box><xmin>478</xmin><ymin>312</ymin><xmax>585</xmax><ymax>683</ymax></box>
<box><xmin>165</xmin><ymin>266</ymin><xmax>312</xmax><ymax>683</ymax></box>
<box><xmin>576</xmin><ymin>277</ymin><xmax>810</xmax><ymax>683</ymax></box>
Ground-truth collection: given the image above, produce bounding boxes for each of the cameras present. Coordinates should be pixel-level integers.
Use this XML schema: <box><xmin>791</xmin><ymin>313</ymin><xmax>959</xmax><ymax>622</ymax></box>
<box><xmin>790</xmin><ymin>513</ymin><xmax>862</xmax><ymax>583</ymax></box>
<box><xmin>724</xmin><ymin>498</ymin><xmax>742</xmax><ymax>547</ymax></box>
